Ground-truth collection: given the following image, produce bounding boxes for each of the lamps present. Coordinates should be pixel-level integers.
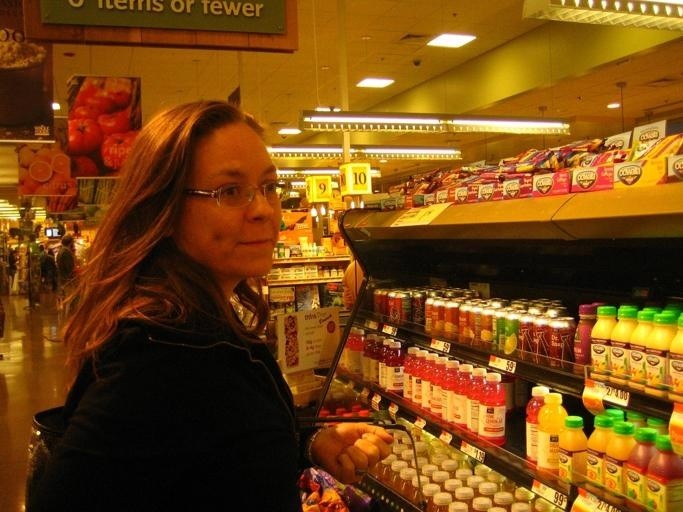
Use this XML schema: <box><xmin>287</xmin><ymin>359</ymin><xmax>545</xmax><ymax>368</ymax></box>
<box><xmin>264</xmin><ymin>143</ymin><xmax>463</xmax><ymax>161</ymax></box>
<box><xmin>520</xmin><ymin>1</ymin><xmax>682</xmax><ymax>33</ymax></box>
<box><xmin>276</xmin><ymin>166</ymin><xmax>381</xmax><ymax>180</ymax></box>
<box><xmin>300</xmin><ymin>110</ymin><xmax>572</xmax><ymax>137</ymax></box>
<box><xmin>278</xmin><ymin>181</ymin><xmax>339</xmax><ymax>190</ymax></box>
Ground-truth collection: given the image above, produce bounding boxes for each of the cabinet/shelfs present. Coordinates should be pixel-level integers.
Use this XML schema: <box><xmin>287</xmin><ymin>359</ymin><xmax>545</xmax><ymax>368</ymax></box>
<box><xmin>309</xmin><ymin>180</ymin><xmax>683</xmax><ymax>512</ymax></box>
<box><xmin>228</xmin><ymin>254</ymin><xmax>354</xmax><ymax>345</ymax></box>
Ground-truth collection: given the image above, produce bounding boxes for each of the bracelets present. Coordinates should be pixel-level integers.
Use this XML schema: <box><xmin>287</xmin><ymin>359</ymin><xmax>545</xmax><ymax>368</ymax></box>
<box><xmin>305</xmin><ymin>427</ymin><xmax>325</xmax><ymax>467</ymax></box>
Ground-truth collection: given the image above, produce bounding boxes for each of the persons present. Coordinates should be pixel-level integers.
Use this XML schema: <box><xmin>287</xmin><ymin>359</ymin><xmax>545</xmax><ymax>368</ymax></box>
<box><xmin>57</xmin><ymin>237</ymin><xmax>77</xmax><ymax>313</ymax></box>
<box><xmin>25</xmin><ymin>97</ymin><xmax>395</xmax><ymax>510</ymax></box>
<box><xmin>46</xmin><ymin>249</ymin><xmax>58</xmax><ymax>290</ymax></box>
<box><xmin>4</xmin><ymin>251</ymin><xmax>20</xmax><ymax>289</ymax></box>
<box><xmin>39</xmin><ymin>245</ymin><xmax>47</xmax><ymax>284</ymax></box>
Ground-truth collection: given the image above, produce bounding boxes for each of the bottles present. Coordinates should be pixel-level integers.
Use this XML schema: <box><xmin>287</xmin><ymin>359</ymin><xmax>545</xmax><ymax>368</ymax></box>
<box><xmin>272</xmin><ymin>235</ymin><xmax>345</xmax><ymax>279</ymax></box>
<box><xmin>316</xmin><ymin>300</ymin><xmax>683</xmax><ymax>512</ymax></box>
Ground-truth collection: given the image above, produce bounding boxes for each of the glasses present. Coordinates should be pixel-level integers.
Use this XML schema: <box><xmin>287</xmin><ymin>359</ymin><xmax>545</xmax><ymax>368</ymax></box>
<box><xmin>187</xmin><ymin>181</ymin><xmax>290</xmax><ymax>209</ymax></box>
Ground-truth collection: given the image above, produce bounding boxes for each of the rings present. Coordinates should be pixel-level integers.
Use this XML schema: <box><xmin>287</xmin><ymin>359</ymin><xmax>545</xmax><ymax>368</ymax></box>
<box><xmin>356</xmin><ymin>468</ymin><xmax>367</xmax><ymax>476</ymax></box>
